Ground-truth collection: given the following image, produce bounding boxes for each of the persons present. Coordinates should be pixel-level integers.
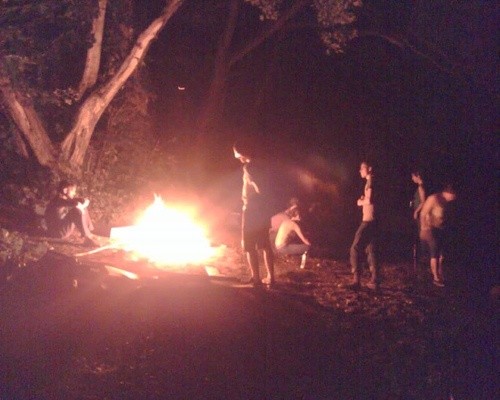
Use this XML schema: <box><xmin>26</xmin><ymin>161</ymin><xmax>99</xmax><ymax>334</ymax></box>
<box><xmin>44</xmin><ymin>178</ymin><xmax>102</xmax><ymax>247</ymax></box>
<box><xmin>277</xmin><ymin>207</ymin><xmax>313</xmax><ymax>271</ymax></box>
<box><xmin>340</xmin><ymin>158</ymin><xmax>387</xmax><ymax>288</ymax></box>
<box><xmin>269</xmin><ymin>198</ymin><xmax>302</xmax><ymax>258</ymax></box>
<box><xmin>407</xmin><ymin>171</ymin><xmax>431</xmax><ymax>278</ymax></box>
<box><xmin>233</xmin><ymin>136</ymin><xmax>275</xmax><ymax>287</ymax></box>
<box><xmin>418</xmin><ymin>185</ymin><xmax>457</xmax><ymax>288</ymax></box>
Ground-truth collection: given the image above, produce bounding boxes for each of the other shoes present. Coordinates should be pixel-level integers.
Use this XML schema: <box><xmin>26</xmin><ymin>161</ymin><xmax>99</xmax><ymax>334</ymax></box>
<box><xmin>433</xmin><ymin>278</ymin><xmax>448</xmax><ymax>288</ymax></box>
<box><xmin>337</xmin><ymin>282</ymin><xmax>360</xmax><ymax>290</ymax></box>
<box><xmin>261</xmin><ymin>277</ymin><xmax>274</xmax><ymax>289</ymax></box>
<box><xmin>360</xmin><ymin>281</ymin><xmax>378</xmax><ymax>291</ymax></box>
<box><xmin>241</xmin><ymin>277</ymin><xmax>262</xmax><ymax>289</ymax></box>
<box><xmin>82</xmin><ymin>235</ymin><xmax>101</xmax><ymax>249</ymax></box>
